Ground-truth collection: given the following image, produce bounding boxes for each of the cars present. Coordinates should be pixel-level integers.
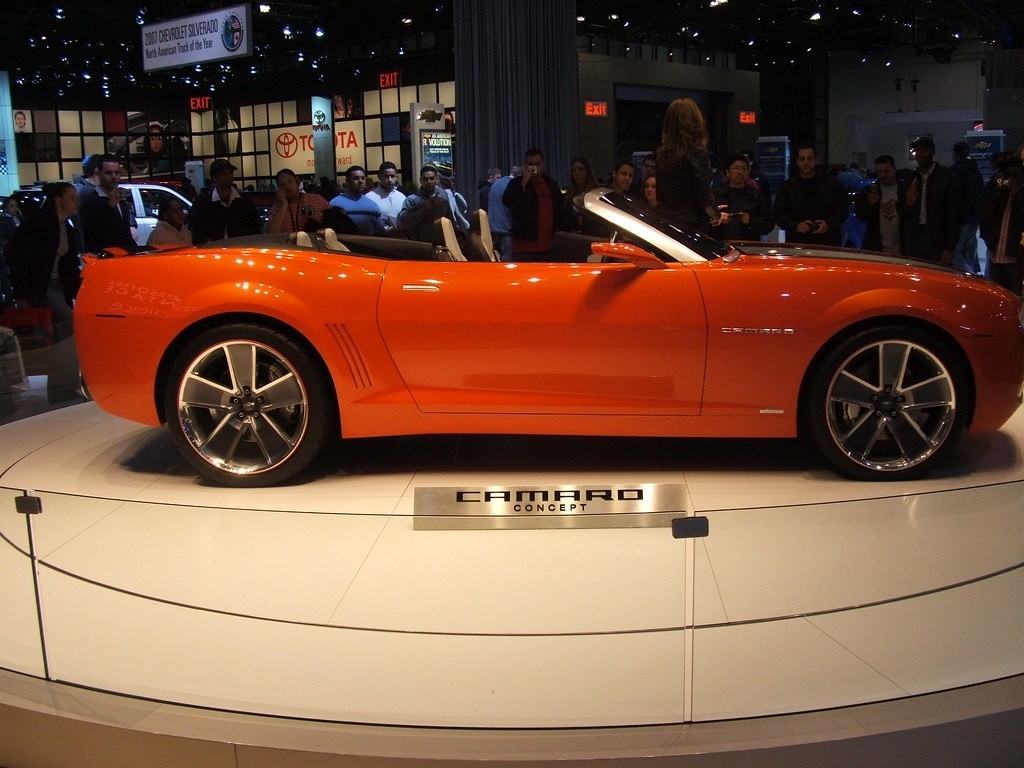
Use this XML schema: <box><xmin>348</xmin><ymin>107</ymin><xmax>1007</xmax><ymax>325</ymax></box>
<box><xmin>0</xmin><ymin>178</ymin><xmax>195</xmax><ymax>248</ymax></box>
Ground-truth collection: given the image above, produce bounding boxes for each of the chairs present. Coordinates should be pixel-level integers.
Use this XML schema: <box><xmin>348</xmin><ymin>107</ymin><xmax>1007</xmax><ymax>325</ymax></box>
<box><xmin>286</xmin><ymin>227</ymin><xmax>352</xmax><ymax>253</ymax></box>
<box><xmin>467</xmin><ymin>208</ymin><xmax>503</xmax><ymax>262</ymax></box>
<box><xmin>431</xmin><ymin>216</ymin><xmax>468</xmax><ymax>262</ymax></box>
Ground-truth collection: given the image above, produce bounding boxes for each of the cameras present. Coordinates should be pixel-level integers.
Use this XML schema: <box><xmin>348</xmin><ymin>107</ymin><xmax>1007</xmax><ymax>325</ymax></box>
<box><xmin>997</xmin><ymin>176</ymin><xmax>1010</xmax><ymax>187</ymax></box>
<box><xmin>806</xmin><ymin>222</ymin><xmax>822</xmax><ymax>230</ymax></box>
<box><xmin>728</xmin><ymin>213</ymin><xmax>743</xmax><ymax>219</ymax></box>
<box><xmin>119</xmin><ymin>190</ymin><xmax>131</xmax><ymax>197</ymax></box>
<box><xmin>529</xmin><ymin>166</ymin><xmax>538</xmax><ymax>173</ymax></box>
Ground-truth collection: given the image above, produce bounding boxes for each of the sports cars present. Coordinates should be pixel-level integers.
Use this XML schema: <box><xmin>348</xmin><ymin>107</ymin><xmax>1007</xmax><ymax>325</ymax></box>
<box><xmin>73</xmin><ymin>185</ymin><xmax>1024</xmax><ymax>488</ymax></box>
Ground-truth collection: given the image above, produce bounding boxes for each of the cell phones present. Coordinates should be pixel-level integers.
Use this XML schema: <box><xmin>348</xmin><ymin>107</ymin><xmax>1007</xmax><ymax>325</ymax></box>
<box><xmin>870</xmin><ymin>184</ymin><xmax>877</xmax><ymax>195</ymax></box>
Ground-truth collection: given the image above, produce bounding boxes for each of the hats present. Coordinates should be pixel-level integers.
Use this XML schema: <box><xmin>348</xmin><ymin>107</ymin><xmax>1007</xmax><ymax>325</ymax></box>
<box><xmin>910</xmin><ymin>137</ymin><xmax>936</xmax><ymax>150</ymax></box>
<box><xmin>211</xmin><ymin>159</ymin><xmax>238</xmax><ymax>173</ymax></box>
<box><xmin>950</xmin><ymin>141</ymin><xmax>969</xmax><ymax>155</ymax></box>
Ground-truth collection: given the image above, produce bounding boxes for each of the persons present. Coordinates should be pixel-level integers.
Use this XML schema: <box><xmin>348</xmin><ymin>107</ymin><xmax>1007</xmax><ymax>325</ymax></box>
<box><xmin>14</xmin><ymin>112</ymin><xmax>31</xmax><ymax>133</ymax></box>
<box><xmin>214</xmin><ymin>100</ymin><xmax>239</xmax><ymax>156</ymax></box>
<box><xmin>444</xmin><ymin>108</ymin><xmax>456</xmax><ymax>141</ymax></box>
<box><xmin>138</xmin><ymin>124</ymin><xmax>177</xmax><ymax>174</ymax></box>
<box><xmin>332</xmin><ymin>92</ymin><xmax>361</xmax><ymax>118</ymax></box>
<box><xmin>0</xmin><ymin>136</ymin><xmax>1024</xmax><ymax>402</ymax></box>
<box><xmin>400</xmin><ymin>114</ymin><xmax>411</xmax><ymax>139</ymax></box>
<box><xmin>654</xmin><ymin>98</ymin><xmax>723</xmax><ymax>263</ymax></box>
